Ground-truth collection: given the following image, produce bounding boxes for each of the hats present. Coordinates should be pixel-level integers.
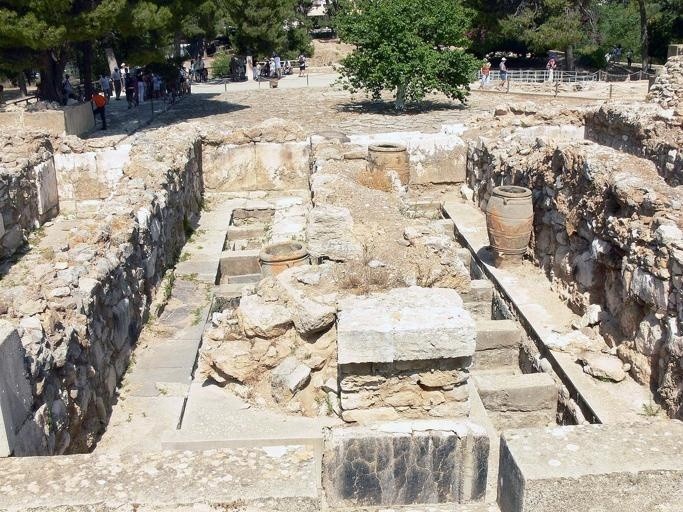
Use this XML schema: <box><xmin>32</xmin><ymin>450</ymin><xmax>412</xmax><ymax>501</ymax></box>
<box><xmin>501</xmin><ymin>57</ymin><xmax>507</xmax><ymax>61</ymax></box>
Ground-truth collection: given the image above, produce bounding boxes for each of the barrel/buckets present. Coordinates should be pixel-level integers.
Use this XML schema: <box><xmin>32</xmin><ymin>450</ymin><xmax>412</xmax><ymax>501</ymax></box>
<box><xmin>368</xmin><ymin>143</ymin><xmax>410</xmax><ymax>190</ymax></box>
<box><xmin>484</xmin><ymin>185</ymin><xmax>534</xmax><ymax>252</ymax></box>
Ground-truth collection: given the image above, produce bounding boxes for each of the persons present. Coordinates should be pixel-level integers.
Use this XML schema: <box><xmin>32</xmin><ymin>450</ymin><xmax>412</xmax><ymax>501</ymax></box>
<box><xmin>626</xmin><ymin>50</ymin><xmax>633</xmax><ymax>67</ymax></box>
<box><xmin>477</xmin><ymin>62</ymin><xmax>492</xmax><ymax>89</ymax></box>
<box><xmin>605</xmin><ymin>52</ymin><xmax>612</xmax><ymax>63</ymax></box>
<box><xmin>196</xmin><ymin>56</ymin><xmax>204</xmax><ymax>82</ymax></box>
<box><xmin>190</xmin><ymin>60</ymin><xmax>196</xmax><ymax>81</ymax></box>
<box><xmin>253</xmin><ymin>53</ymin><xmax>307</xmax><ymax>81</ymax></box>
<box><xmin>62</xmin><ymin>62</ymin><xmax>193</xmax><ymax>132</ymax></box>
<box><xmin>494</xmin><ymin>57</ymin><xmax>508</xmax><ymax>91</ymax></box>
<box><xmin>483</xmin><ymin>58</ymin><xmax>491</xmax><ymax>83</ymax></box>
<box><xmin>614</xmin><ymin>44</ymin><xmax>623</xmax><ymax>65</ymax></box>
<box><xmin>545</xmin><ymin>59</ymin><xmax>556</xmax><ymax>82</ymax></box>
